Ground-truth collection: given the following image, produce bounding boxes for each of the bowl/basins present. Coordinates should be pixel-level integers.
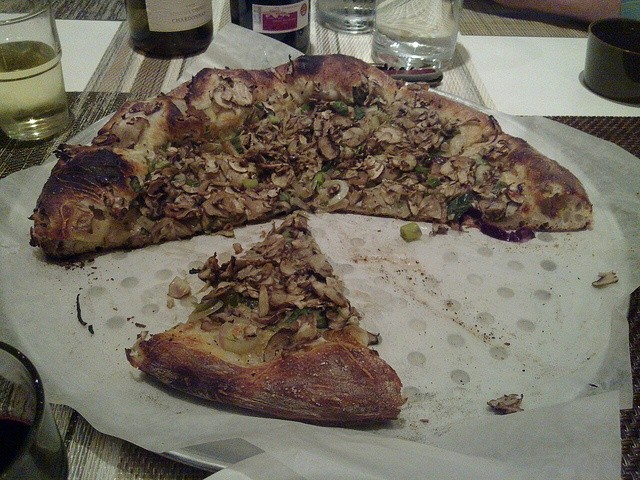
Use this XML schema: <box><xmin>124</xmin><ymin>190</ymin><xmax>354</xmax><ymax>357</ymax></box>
<box><xmin>584</xmin><ymin>18</ymin><xmax>640</xmax><ymax>104</ymax></box>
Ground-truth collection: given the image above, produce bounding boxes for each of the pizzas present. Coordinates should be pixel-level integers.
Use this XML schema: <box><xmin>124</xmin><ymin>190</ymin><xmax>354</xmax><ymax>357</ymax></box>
<box><xmin>125</xmin><ymin>210</ymin><xmax>409</xmax><ymax>425</ymax></box>
<box><xmin>28</xmin><ymin>53</ymin><xmax>593</xmax><ymax>261</ymax></box>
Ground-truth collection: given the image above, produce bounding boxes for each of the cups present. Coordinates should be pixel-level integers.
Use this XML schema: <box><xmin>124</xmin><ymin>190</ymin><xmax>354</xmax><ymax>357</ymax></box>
<box><xmin>1</xmin><ymin>342</ymin><xmax>70</xmax><ymax>480</ymax></box>
<box><xmin>313</xmin><ymin>1</ymin><xmax>376</xmax><ymax>35</ymax></box>
<box><xmin>372</xmin><ymin>1</ymin><xmax>463</xmax><ymax>76</ymax></box>
<box><xmin>0</xmin><ymin>0</ymin><xmax>69</xmax><ymax>143</ymax></box>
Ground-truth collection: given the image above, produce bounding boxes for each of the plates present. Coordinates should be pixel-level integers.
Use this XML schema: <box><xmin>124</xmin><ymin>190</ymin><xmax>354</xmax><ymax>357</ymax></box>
<box><xmin>200</xmin><ymin>438</ymin><xmax>507</xmax><ymax>480</ymax></box>
<box><xmin>0</xmin><ymin>85</ymin><xmax>625</xmax><ymax>473</ymax></box>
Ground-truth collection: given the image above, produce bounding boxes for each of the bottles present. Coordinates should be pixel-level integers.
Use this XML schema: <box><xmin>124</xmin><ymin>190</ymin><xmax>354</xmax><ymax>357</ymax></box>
<box><xmin>124</xmin><ymin>0</ymin><xmax>213</xmax><ymax>59</ymax></box>
<box><xmin>228</xmin><ymin>1</ymin><xmax>311</xmax><ymax>55</ymax></box>
